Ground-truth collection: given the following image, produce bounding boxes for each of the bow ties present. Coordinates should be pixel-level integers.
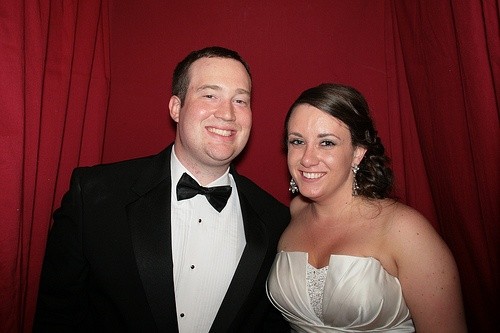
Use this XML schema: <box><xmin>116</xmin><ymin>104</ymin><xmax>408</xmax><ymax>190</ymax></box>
<box><xmin>175</xmin><ymin>171</ymin><xmax>233</xmax><ymax>213</ymax></box>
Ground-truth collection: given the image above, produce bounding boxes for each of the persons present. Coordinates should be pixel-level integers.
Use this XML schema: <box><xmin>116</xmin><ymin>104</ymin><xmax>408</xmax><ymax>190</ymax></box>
<box><xmin>31</xmin><ymin>45</ymin><xmax>291</xmax><ymax>333</ymax></box>
<box><xmin>265</xmin><ymin>84</ymin><xmax>469</xmax><ymax>333</ymax></box>
